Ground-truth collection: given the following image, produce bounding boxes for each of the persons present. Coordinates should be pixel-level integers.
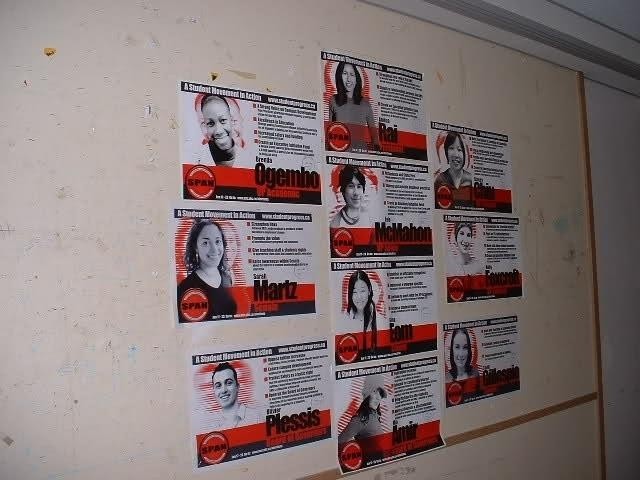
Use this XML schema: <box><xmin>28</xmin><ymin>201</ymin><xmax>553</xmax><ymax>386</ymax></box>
<box><xmin>337</xmin><ymin>271</ymin><xmax>388</xmax><ymax>331</ymax></box>
<box><xmin>177</xmin><ymin>221</ymin><xmax>237</xmax><ymax>323</ymax></box>
<box><xmin>337</xmin><ymin>374</ymin><xmax>387</xmax><ymax>444</ymax></box>
<box><xmin>436</xmin><ymin>131</ymin><xmax>473</xmax><ymax>210</ymax></box>
<box><xmin>211</xmin><ymin>363</ymin><xmax>256</xmax><ymax>431</ymax></box>
<box><xmin>199</xmin><ymin>97</ymin><xmax>249</xmax><ymax>168</ymax></box>
<box><xmin>445</xmin><ymin>329</ymin><xmax>481</xmax><ymax>393</ymax></box>
<box><xmin>453</xmin><ymin>223</ymin><xmax>482</xmax><ymax>275</ymax></box>
<box><xmin>328</xmin><ymin>64</ymin><xmax>382</xmax><ymax>153</ymax></box>
<box><xmin>331</xmin><ymin>168</ymin><xmax>372</xmax><ymax>229</ymax></box>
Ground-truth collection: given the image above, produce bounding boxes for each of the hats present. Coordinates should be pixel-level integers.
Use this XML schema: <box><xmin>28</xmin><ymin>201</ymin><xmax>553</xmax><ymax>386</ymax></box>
<box><xmin>362</xmin><ymin>374</ymin><xmax>388</xmax><ymax>401</ymax></box>
